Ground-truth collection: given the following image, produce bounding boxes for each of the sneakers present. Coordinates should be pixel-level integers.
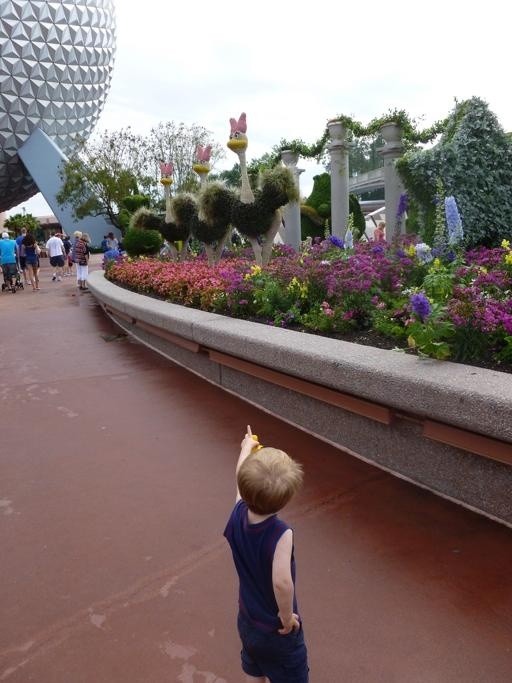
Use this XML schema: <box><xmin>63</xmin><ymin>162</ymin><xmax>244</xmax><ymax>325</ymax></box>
<box><xmin>51</xmin><ymin>276</ymin><xmax>62</xmax><ymax>282</ymax></box>
<box><xmin>78</xmin><ymin>286</ymin><xmax>89</xmax><ymax>291</ymax></box>
<box><xmin>32</xmin><ymin>287</ymin><xmax>41</xmax><ymax>292</ymax></box>
<box><xmin>8</xmin><ymin>284</ymin><xmax>17</xmax><ymax>293</ymax></box>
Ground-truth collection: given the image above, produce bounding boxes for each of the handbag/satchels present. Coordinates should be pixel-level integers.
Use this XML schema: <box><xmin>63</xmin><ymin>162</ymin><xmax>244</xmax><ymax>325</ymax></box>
<box><xmin>79</xmin><ymin>260</ymin><xmax>87</xmax><ymax>266</ymax></box>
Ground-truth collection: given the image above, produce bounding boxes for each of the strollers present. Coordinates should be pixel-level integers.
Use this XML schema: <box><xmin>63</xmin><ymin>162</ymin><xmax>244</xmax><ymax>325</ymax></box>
<box><xmin>0</xmin><ymin>256</ymin><xmax>25</xmax><ymax>292</ymax></box>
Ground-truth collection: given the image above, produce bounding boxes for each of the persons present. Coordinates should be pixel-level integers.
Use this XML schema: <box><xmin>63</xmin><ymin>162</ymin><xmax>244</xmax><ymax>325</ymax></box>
<box><xmin>374</xmin><ymin>222</ymin><xmax>386</xmax><ymax>241</ymax></box>
<box><xmin>225</xmin><ymin>425</ymin><xmax>309</xmax><ymax>683</ymax></box>
<box><xmin>0</xmin><ymin>224</ymin><xmax>127</xmax><ymax>294</ymax></box>
